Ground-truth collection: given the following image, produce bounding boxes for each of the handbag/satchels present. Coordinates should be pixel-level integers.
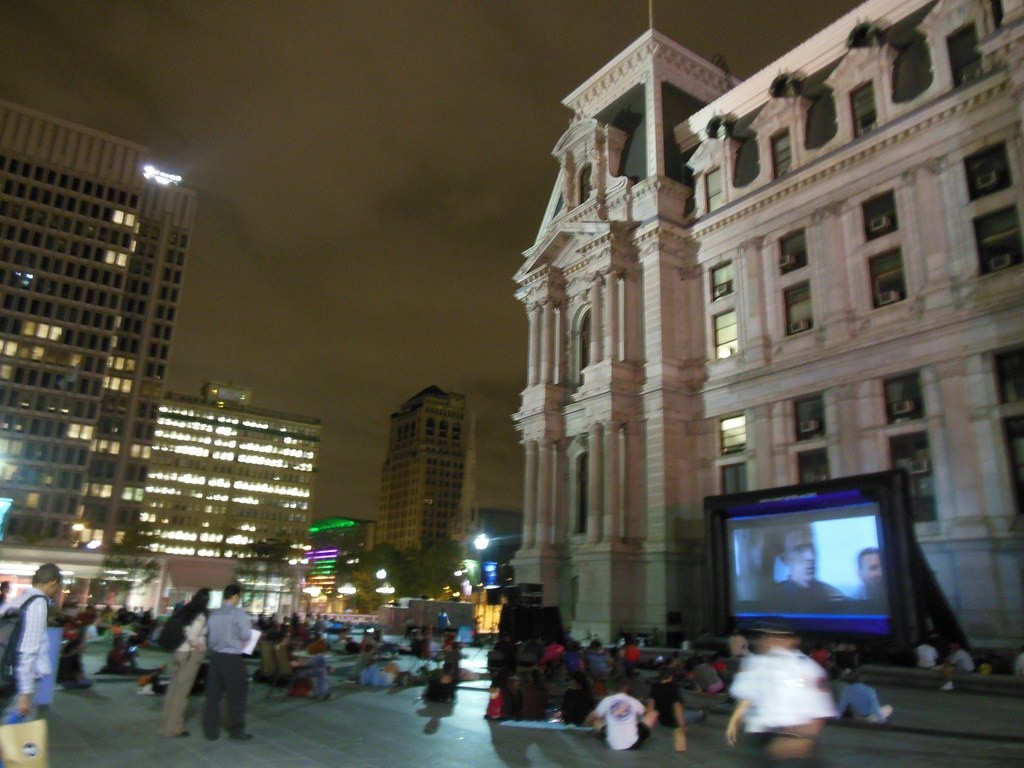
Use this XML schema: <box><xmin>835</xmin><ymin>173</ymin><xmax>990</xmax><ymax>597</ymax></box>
<box><xmin>0</xmin><ymin>708</ymin><xmax>47</xmax><ymax>768</ymax></box>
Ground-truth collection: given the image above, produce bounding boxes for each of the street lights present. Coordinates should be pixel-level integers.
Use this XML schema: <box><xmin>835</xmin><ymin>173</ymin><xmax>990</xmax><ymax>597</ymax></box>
<box><xmin>472</xmin><ymin>532</ymin><xmax>489</xmax><ymax>606</ymax></box>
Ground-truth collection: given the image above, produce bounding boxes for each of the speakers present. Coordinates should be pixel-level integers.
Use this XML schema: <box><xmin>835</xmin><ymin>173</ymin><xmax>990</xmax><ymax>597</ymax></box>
<box><xmin>485</xmin><ymin>584</ymin><xmax>501</xmax><ymax>605</ymax></box>
<box><xmin>506</xmin><ymin>585</ymin><xmax>522</xmax><ymax>605</ymax></box>
<box><xmin>666</xmin><ymin>611</ymin><xmax>682</xmax><ymax>626</ymax></box>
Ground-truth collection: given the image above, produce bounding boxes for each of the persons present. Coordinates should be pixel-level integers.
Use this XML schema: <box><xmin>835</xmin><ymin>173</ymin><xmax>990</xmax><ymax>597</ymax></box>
<box><xmin>776</xmin><ymin>520</ymin><xmax>845</xmax><ymax>609</ymax></box>
<box><xmin>855</xmin><ymin>548</ymin><xmax>885</xmax><ymax>606</ymax></box>
<box><xmin>1</xmin><ymin>509</ymin><xmax>1024</xmax><ymax>768</ymax></box>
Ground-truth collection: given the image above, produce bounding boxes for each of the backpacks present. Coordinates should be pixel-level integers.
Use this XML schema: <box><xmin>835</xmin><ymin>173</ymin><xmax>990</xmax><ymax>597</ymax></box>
<box><xmin>159</xmin><ymin>609</ymin><xmax>209</xmax><ymax>650</ymax></box>
<box><xmin>0</xmin><ymin>594</ymin><xmax>48</xmax><ymax>698</ymax></box>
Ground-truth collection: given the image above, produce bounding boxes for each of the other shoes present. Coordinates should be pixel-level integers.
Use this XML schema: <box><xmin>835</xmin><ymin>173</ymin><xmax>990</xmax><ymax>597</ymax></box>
<box><xmin>179</xmin><ymin>731</ymin><xmax>191</xmax><ymax>736</ymax></box>
<box><xmin>700</xmin><ymin>704</ymin><xmax>709</xmax><ymax>720</ymax></box>
<box><xmin>236</xmin><ymin>733</ymin><xmax>253</xmax><ymax>740</ymax></box>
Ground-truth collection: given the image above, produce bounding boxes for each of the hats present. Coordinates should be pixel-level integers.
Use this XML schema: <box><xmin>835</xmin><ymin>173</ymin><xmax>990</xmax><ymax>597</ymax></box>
<box><xmin>740</xmin><ymin>621</ymin><xmax>799</xmax><ymax>638</ymax></box>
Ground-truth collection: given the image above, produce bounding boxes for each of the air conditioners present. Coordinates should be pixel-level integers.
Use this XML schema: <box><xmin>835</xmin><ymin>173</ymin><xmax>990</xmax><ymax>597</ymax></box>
<box><xmin>879</xmin><ymin>291</ymin><xmax>899</xmax><ymax>305</ymax></box>
<box><xmin>992</xmin><ymin>254</ymin><xmax>1011</xmax><ymax>270</ymax></box>
<box><xmin>893</xmin><ymin>401</ymin><xmax>914</xmax><ymax>415</ymax></box>
<box><xmin>910</xmin><ymin>460</ymin><xmax>927</xmax><ymax>473</ymax></box>
<box><xmin>977</xmin><ymin>172</ymin><xmax>995</xmax><ymax>188</ymax></box>
<box><xmin>872</xmin><ymin>217</ymin><xmax>890</xmax><ymax>231</ymax></box>
<box><xmin>791</xmin><ymin>320</ymin><xmax>808</xmax><ymax>333</ymax></box>
<box><xmin>801</xmin><ymin>420</ymin><xmax>818</xmax><ymax>432</ymax></box>
<box><xmin>779</xmin><ymin>254</ymin><xmax>795</xmax><ymax>266</ymax></box>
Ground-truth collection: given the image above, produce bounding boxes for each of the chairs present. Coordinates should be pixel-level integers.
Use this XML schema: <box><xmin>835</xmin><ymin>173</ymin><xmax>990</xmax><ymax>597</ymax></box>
<box><xmin>261</xmin><ymin>642</ymin><xmax>317</xmax><ymax>703</ymax></box>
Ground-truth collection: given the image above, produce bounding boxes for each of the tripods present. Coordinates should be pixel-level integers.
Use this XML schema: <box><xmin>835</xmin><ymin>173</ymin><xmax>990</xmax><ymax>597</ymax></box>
<box><xmin>471</xmin><ymin>605</ymin><xmax>496</xmax><ymax>661</ymax></box>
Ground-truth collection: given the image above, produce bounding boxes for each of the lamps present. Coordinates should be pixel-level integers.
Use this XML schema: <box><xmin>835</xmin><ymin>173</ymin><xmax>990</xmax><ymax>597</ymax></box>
<box><xmin>769</xmin><ymin>73</ymin><xmax>799</xmax><ymax>97</ymax></box>
<box><xmin>846</xmin><ymin>22</ymin><xmax>879</xmax><ymax>48</ymax></box>
<box><xmin>705</xmin><ymin>115</ymin><xmax>732</xmax><ymax>138</ymax></box>
